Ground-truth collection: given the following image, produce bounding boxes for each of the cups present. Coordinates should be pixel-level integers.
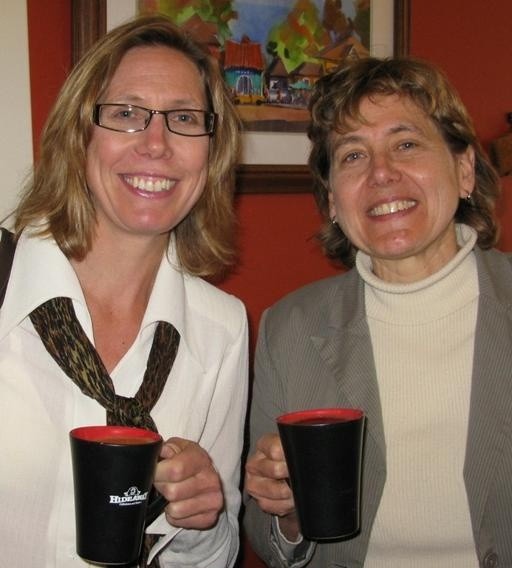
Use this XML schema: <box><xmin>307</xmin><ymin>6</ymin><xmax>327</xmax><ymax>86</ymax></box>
<box><xmin>69</xmin><ymin>425</ymin><xmax>171</xmax><ymax>567</ymax></box>
<box><xmin>276</xmin><ymin>409</ymin><xmax>368</xmax><ymax>545</ymax></box>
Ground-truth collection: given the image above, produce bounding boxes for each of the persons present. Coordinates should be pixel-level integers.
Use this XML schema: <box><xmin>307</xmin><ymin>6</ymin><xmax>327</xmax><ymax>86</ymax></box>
<box><xmin>0</xmin><ymin>14</ymin><xmax>251</xmax><ymax>568</ymax></box>
<box><xmin>239</xmin><ymin>53</ymin><xmax>512</xmax><ymax>567</ymax></box>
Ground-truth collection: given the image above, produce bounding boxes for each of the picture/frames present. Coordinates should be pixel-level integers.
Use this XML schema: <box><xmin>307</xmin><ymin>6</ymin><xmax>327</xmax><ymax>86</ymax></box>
<box><xmin>68</xmin><ymin>1</ymin><xmax>412</xmax><ymax>197</ymax></box>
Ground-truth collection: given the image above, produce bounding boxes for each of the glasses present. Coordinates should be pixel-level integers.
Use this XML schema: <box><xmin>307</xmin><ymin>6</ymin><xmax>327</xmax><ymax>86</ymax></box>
<box><xmin>92</xmin><ymin>100</ymin><xmax>219</xmax><ymax>138</ymax></box>
